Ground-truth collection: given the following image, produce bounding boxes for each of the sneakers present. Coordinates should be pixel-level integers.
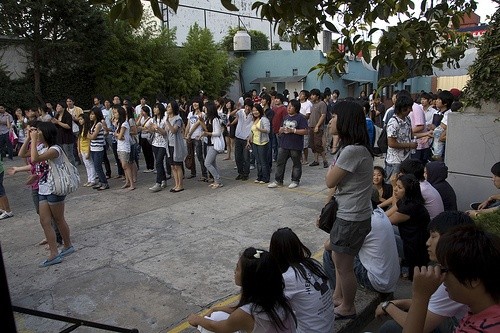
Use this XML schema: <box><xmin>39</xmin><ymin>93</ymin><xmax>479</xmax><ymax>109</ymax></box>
<box><xmin>289</xmin><ymin>181</ymin><xmax>299</xmax><ymax>188</ymax></box>
<box><xmin>0</xmin><ymin>211</ymin><xmax>14</xmax><ymax>220</ymax></box>
<box><xmin>268</xmin><ymin>182</ymin><xmax>283</xmax><ymax>188</ymax></box>
<box><xmin>323</xmin><ymin>161</ymin><xmax>328</xmax><ymax>168</ymax></box>
<box><xmin>309</xmin><ymin>161</ymin><xmax>319</xmax><ymax>166</ymax></box>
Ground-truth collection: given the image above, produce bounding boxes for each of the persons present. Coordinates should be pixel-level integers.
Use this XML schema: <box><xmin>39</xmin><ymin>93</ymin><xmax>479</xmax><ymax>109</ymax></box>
<box><xmin>269</xmin><ymin>227</ymin><xmax>335</xmax><ymax>333</ymax></box>
<box><xmin>425</xmin><ymin>161</ymin><xmax>457</xmax><ymax>212</ymax></box>
<box><xmin>0</xmin><ymin>98</ymin><xmax>83</xmax><ymax>166</ymax></box>
<box><xmin>223</xmin><ymin>87</ymin><xmax>339</xmax><ymax>189</ymax></box>
<box><xmin>0</xmin><ymin>160</ymin><xmax>13</xmax><ymax>221</ymax></box>
<box><xmin>357</xmin><ymin>89</ymin><xmax>386</xmax><ymax>155</ymax></box>
<box><xmin>322</xmin><ymin>185</ymin><xmax>399</xmax><ymax>292</ymax></box>
<box><xmin>466</xmin><ymin>162</ymin><xmax>500</xmax><ymax>218</ymax></box>
<box><xmin>385</xmin><ymin>173</ymin><xmax>431</xmax><ymax>275</ymax></box>
<box><xmin>77</xmin><ymin>91</ymin><xmax>224</xmax><ymax>193</ymax></box>
<box><xmin>373</xmin><ymin>166</ymin><xmax>392</xmax><ymax>211</ymax></box>
<box><xmin>326</xmin><ymin>100</ymin><xmax>372</xmax><ymax>319</ymax></box>
<box><xmin>380</xmin><ymin>159</ymin><xmax>444</xmax><ymax>220</ymax></box>
<box><xmin>383</xmin><ymin>88</ymin><xmax>465</xmax><ymax>181</ymax></box>
<box><xmin>18</xmin><ymin>122</ymin><xmax>73</xmax><ymax>268</ymax></box>
<box><xmin>361</xmin><ymin>212</ymin><xmax>500</xmax><ymax>333</ymax></box>
<box><xmin>188</xmin><ymin>247</ymin><xmax>298</xmax><ymax>333</ymax></box>
<box><xmin>5</xmin><ymin>121</ymin><xmax>63</xmax><ymax>249</ymax></box>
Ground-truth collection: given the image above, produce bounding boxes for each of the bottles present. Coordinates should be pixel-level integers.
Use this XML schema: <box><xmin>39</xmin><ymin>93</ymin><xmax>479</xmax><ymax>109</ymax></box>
<box><xmin>248</xmin><ymin>146</ymin><xmax>253</xmax><ymax>153</ymax></box>
<box><xmin>410</xmin><ymin>136</ymin><xmax>417</xmax><ymax>155</ymax></box>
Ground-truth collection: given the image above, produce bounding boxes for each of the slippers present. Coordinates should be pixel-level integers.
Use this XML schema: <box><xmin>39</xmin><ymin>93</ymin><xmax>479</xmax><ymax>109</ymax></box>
<box><xmin>334</xmin><ymin>310</ymin><xmax>357</xmax><ymax>319</ymax></box>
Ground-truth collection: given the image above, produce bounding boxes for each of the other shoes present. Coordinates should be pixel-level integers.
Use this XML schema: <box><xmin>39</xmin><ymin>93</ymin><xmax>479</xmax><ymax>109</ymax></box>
<box><xmin>302</xmin><ymin>161</ymin><xmax>309</xmax><ymax>165</ymax></box>
<box><xmin>58</xmin><ymin>246</ymin><xmax>73</xmax><ymax>255</ymax></box>
<box><xmin>39</xmin><ymin>255</ymin><xmax>62</xmax><ymax>267</ymax></box>
<box><xmin>144</xmin><ymin>168</ymin><xmax>224</xmax><ymax>193</ymax></box>
<box><xmin>232</xmin><ymin>157</ymin><xmax>276</xmax><ymax>184</ymax></box>
<box><xmin>83</xmin><ymin>175</ymin><xmax>139</xmax><ymax>190</ymax></box>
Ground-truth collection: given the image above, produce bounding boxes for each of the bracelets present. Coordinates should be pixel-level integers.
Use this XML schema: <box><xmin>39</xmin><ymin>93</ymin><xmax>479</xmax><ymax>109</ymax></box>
<box><xmin>475</xmin><ymin>213</ymin><xmax>478</xmax><ymax>217</ymax></box>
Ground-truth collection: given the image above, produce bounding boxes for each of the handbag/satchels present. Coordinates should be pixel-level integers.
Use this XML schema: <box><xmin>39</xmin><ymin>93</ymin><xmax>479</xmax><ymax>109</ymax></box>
<box><xmin>317</xmin><ymin>196</ymin><xmax>338</xmax><ymax>234</ymax></box>
<box><xmin>72</xmin><ymin>120</ymin><xmax>79</xmax><ymax>134</ymax></box>
<box><xmin>185</xmin><ymin>140</ymin><xmax>194</xmax><ymax>169</ymax></box>
<box><xmin>214</xmin><ymin>135</ymin><xmax>225</xmax><ymax>152</ymax></box>
<box><xmin>130</xmin><ymin>125</ymin><xmax>137</xmax><ymax>135</ymax></box>
<box><xmin>139</xmin><ymin>138</ymin><xmax>149</xmax><ymax>146</ymax></box>
<box><xmin>48</xmin><ymin>146</ymin><xmax>80</xmax><ymax>195</ymax></box>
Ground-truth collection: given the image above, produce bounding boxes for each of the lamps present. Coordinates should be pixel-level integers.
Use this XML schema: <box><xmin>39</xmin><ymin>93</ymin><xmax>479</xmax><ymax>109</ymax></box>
<box><xmin>233</xmin><ymin>16</ymin><xmax>251</xmax><ymax>51</ymax></box>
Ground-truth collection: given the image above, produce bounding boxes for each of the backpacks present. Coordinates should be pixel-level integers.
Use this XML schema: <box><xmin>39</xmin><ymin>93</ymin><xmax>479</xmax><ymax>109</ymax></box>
<box><xmin>377</xmin><ymin>116</ymin><xmax>411</xmax><ymax>152</ymax></box>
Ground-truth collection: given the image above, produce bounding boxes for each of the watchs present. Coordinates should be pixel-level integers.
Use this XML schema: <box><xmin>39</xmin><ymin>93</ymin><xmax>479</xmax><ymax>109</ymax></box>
<box><xmin>382</xmin><ymin>301</ymin><xmax>394</xmax><ymax>313</ymax></box>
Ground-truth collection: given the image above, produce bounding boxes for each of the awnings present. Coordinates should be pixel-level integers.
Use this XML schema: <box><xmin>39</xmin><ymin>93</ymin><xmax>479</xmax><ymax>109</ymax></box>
<box><xmin>250</xmin><ymin>75</ymin><xmax>307</xmax><ymax>84</ymax></box>
<box><xmin>343</xmin><ymin>78</ymin><xmax>373</xmax><ymax>86</ymax></box>
